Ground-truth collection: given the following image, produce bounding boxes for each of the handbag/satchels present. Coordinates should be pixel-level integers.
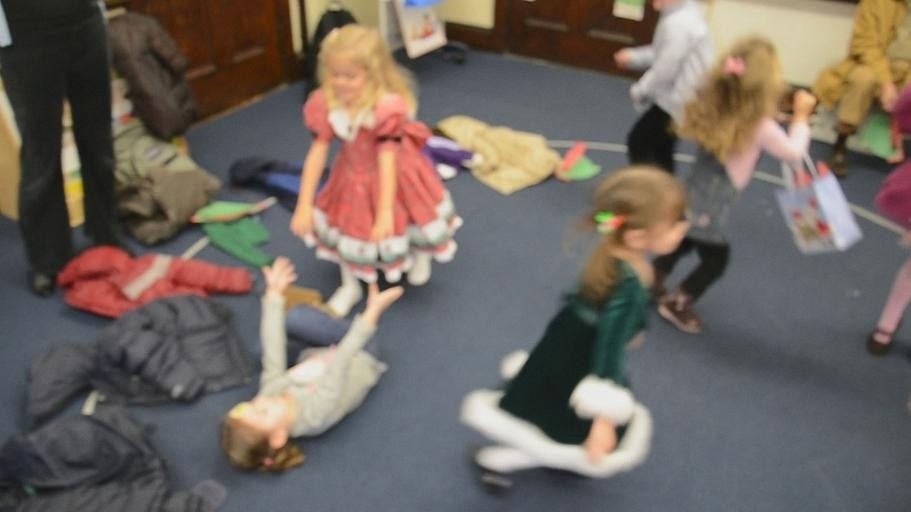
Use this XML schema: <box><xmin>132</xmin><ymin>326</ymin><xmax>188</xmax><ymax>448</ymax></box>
<box><xmin>772</xmin><ymin>143</ymin><xmax>862</xmax><ymax>257</ymax></box>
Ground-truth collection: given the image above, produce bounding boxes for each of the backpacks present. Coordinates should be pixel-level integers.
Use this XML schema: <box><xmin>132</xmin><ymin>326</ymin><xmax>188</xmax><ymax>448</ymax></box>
<box><xmin>304</xmin><ymin>5</ymin><xmax>357</xmax><ymax>96</ymax></box>
<box><xmin>109</xmin><ymin>121</ymin><xmax>222</xmax><ymax>246</ymax></box>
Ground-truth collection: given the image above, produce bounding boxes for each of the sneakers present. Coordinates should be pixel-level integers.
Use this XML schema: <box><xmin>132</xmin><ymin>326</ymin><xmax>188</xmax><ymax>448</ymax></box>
<box><xmin>868</xmin><ymin>328</ymin><xmax>892</xmax><ymax>356</ymax></box>
<box><xmin>654</xmin><ymin>298</ymin><xmax>702</xmax><ymax>335</ymax></box>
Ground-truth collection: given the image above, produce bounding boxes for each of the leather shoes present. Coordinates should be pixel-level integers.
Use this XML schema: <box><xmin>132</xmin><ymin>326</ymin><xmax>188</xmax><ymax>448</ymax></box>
<box><xmin>28</xmin><ymin>263</ymin><xmax>56</xmax><ymax>299</ymax></box>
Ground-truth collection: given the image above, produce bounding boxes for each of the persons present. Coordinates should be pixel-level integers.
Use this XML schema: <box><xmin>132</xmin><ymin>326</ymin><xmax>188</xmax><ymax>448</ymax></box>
<box><xmin>453</xmin><ymin>162</ymin><xmax>691</xmax><ymax>492</ymax></box>
<box><xmin>866</xmin><ymin>84</ymin><xmax>911</xmax><ymax>355</ymax></box>
<box><xmin>0</xmin><ymin>0</ymin><xmax>136</xmax><ymax>298</ymax></box>
<box><xmin>613</xmin><ymin>0</ymin><xmax>719</xmax><ymax>174</ymax></box>
<box><xmin>646</xmin><ymin>36</ymin><xmax>817</xmax><ymax>334</ymax></box>
<box><xmin>288</xmin><ymin>23</ymin><xmax>465</xmax><ymax>320</ymax></box>
<box><xmin>220</xmin><ymin>255</ymin><xmax>405</xmax><ymax>474</ymax></box>
<box><xmin>810</xmin><ymin>0</ymin><xmax>911</xmax><ymax>180</ymax></box>
